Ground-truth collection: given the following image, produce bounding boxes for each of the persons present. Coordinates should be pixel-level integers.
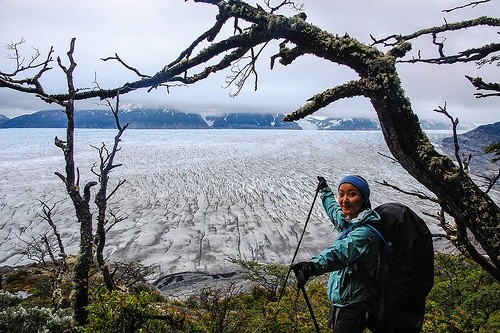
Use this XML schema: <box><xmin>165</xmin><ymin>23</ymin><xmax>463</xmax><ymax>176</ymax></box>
<box><xmin>292</xmin><ymin>175</ymin><xmax>390</xmax><ymax>333</ymax></box>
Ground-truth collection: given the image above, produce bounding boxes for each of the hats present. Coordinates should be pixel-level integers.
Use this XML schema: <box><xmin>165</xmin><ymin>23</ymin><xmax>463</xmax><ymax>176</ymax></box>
<box><xmin>337</xmin><ymin>174</ymin><xmax>371</xmax><ymax>204</ymax></box>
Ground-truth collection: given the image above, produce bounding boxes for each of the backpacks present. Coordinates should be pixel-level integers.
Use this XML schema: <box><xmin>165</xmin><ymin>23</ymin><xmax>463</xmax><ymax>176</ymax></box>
<box><xmin>338</xmin><ymin>202</ymin><xmax>434</xmax><ymax>333</ymax></box>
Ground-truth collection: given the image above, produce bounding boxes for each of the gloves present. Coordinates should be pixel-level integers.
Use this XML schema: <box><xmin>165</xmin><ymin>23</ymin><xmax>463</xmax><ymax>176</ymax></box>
<box><xmin>292</xmin><ymin>261</ymin><xmax>314</xmax><ymax>289</ymax></box>
<box><xmin>315</xmin><ymin>176</ymin><xmax>328</xmax><ymax>190</ymax></box>
